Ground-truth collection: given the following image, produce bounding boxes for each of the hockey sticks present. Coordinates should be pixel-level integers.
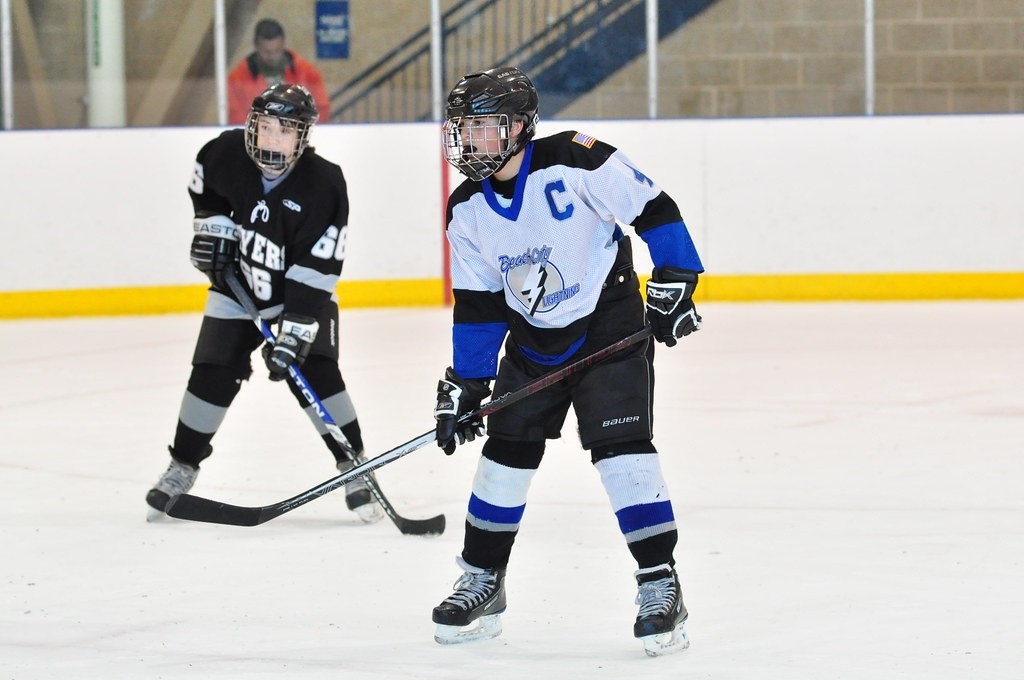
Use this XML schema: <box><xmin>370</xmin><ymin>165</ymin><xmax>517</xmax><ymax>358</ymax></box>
<box><xmin>219</xmin><ymin>266</ymin><xmax>448</xmax><ymax>537</ymax></box>
<box><xmin>166</xmin><ymin>323</ymin><xmax>663</xmax><ymax>527</ymax></box>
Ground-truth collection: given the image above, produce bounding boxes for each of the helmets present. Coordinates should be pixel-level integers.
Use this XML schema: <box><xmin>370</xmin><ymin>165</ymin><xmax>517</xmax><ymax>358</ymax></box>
<box><xmin>244</xmin><ymin>83</ymin><xmax>320</xmax><ymax>170</ymax></box>
<box><xmin>442</xmin><ymin>66</ymin><xmax>538</xmax><ymax>182</ymax></box>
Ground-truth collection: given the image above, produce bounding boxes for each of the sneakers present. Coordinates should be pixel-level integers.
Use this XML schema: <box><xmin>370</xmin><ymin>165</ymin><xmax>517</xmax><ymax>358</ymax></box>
<box><xmin>432</xmin><ymin>555</ymin><xmax>507</xmax><ymax>644</ymax></box>
<box><xmin>144</xmin><ymin>445</ymin><xmax>213</xmax><ymax>522</ymax></box>
<box><xmin>632</xmin><ymin>558</ymin><xmax>688</xmax><ymax>656</ymax></box>
<box><xmin>335</xmin><ymin>449</ymin><xmax>382</xmax><ymax>524</ymax></box>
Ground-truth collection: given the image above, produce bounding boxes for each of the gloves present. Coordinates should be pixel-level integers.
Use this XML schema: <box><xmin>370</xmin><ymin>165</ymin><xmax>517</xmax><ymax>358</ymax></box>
<box><xmin>434</xmin><ymin>366</ymin><xmax>492</xmax><ymax>455</ymax></box>
<box><xmin>261</xmin><ymin>313</ymin><xmax>319</xmax><ymax>382</ymax></box>
<box><xmin>642</xmin><ymin>264</ymin><xmax>700</xmax><ymax>346</ymax></box>
<box><xmin>189</xmin><ymin>208</ymin><xmax>243</xmax><ymax>295</ymax></box>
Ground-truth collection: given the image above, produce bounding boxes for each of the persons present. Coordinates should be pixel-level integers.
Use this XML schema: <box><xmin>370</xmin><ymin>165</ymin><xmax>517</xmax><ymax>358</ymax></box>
<box><xmin>430</xmin><ymin>65</ymin><xmax>705</xmax><ymax>655</ymax></box>
<box><xmin>142</xmin><ymin>81</ymin><xmax>381</xmax><ymax>523</ymax></box>
<box><xmin>228</xmin><ymin>18</ymin><xmax>333</xmax><ymax>126</ymax></box>
